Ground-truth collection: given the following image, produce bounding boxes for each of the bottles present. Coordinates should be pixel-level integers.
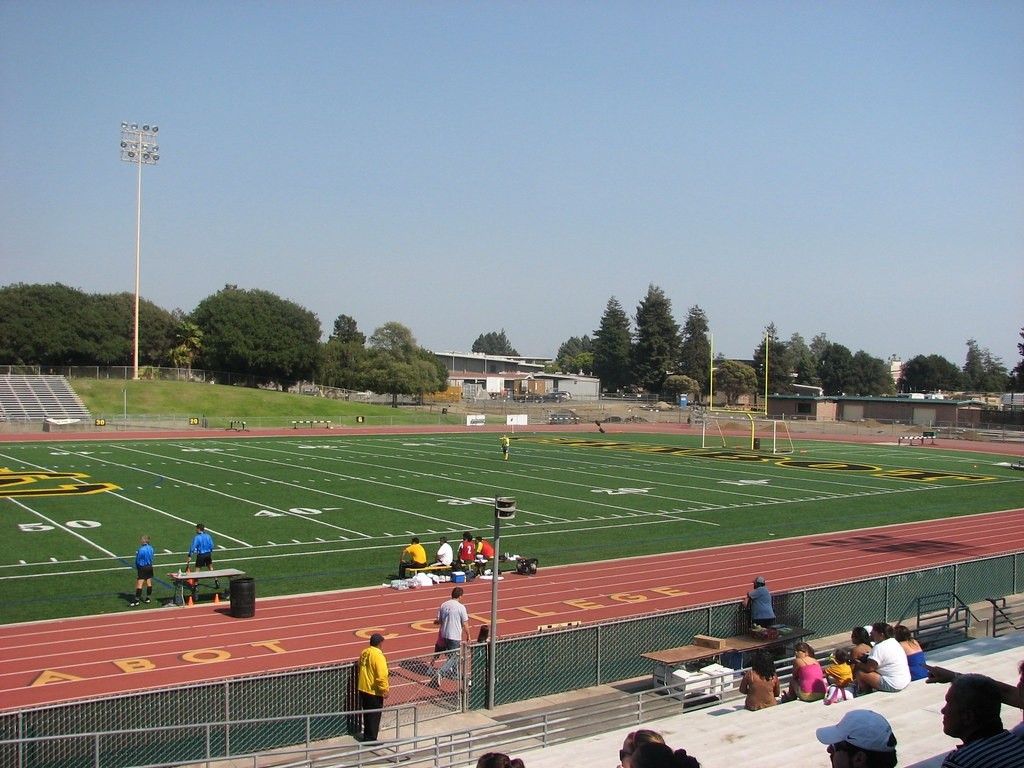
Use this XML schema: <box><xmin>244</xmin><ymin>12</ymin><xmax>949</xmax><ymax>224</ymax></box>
<box><xmin>177</xmin><ymin>569</ymin><xmax>182</xmax><ymax>578</ymax></box>
<box><xmin>829</xmin><ymin>652</ymin><xmax>833</xmax><ymax>661</ymax></box>
<box><xmin>846</xmin><ymin>655</ymin><xmax>869</xmax><ymax>665</ymax></box>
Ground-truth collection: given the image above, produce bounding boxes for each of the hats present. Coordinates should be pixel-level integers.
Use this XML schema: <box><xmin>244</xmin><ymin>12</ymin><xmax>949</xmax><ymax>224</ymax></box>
<box><xmin>752</xmin><ymin>576</ymin><xmax>766</xmax><ymax>585</ymax></box>
<box><xmin>370</xmin><ymin>634</ymin><xmax>384</xmax><ymax>645</ymax></box>
<box><xmin>816</xmin><ymin>710</ymin><xmax>896</xmax><ymax>752</ymax></box>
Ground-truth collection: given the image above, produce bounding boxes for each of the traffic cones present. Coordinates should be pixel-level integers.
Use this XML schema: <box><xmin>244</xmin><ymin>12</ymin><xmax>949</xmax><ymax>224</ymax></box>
<box><xmin>214</xmin><ymin>594</ymin><xmax>220</xmax><ymax>604</ymax></box>
<box><xmin>188</xmin><ymin>596</ymin><xmax>194</xmax><ymax>606</ymax></box>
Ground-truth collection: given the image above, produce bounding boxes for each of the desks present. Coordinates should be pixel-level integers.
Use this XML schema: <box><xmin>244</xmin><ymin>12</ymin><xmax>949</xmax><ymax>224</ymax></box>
<box><xmin>166</xmin><ymin>568</ymin><xmax>246</xmax><ymax>607</ymax></box>
<box><xmin>640</xmin><ymin>623</ymin><xmax>816</xmax><ymax>701</ymax></box>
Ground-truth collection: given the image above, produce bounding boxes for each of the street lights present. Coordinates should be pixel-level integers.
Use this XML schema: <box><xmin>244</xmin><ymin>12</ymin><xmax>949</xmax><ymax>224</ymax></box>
<box><xmin>121</xmin><ymin>121</ymin><xmax>160</xmax><ymax>379</ymax></box>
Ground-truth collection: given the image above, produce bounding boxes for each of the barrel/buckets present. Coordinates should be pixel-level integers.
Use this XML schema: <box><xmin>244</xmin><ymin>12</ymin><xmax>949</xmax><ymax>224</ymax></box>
<box><xmin>230</xmin><ymin>577</ymin><xmax>255</xmax><ymax>617</ymax></box>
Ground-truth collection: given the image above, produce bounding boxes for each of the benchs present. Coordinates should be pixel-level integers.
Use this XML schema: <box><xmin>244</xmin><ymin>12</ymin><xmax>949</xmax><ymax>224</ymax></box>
<box><xmin>0</xmin><ymin>375</ymin><xmax>93</xmax><ymax>423</ymax></box>
<box><xmin>405</xmin><ymin>557</ymin><xmax>528</xmax><ymax>576</ymax></box>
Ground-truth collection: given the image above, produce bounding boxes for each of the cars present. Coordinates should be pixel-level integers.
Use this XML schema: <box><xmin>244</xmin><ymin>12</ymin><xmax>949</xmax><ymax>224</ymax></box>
<box><xmin>517</xmin><ymin>392</ymin><xmax>545</xmax><ymax>404</ymax></box>
<box><xmin>601</xmin><ymin>416</ymin><xmax>647</xmax><ymax>423</ymax></box>
<box><xmin>543</xmin><ymin>391</ymin><xmax>572</xmax><ymax>403</ymax></box>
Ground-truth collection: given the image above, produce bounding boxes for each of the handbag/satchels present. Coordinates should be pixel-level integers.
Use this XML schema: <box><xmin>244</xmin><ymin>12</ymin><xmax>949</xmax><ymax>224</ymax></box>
<box><xmin>824</xmin><ymin>684</ymin><xmax>854</xmax><ymax>702</ymax></box>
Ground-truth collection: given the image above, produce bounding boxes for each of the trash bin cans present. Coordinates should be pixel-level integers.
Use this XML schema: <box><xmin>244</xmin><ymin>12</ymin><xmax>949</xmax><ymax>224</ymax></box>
<box><xmin>231</xmin><ymin>577</ymin><xmax>256</xmax><ymax>616</ymax></box>
<box><xmin>679</xmin><ymin>394</ymin><xmax>687</xmax><ymax>407</ymax></box>
<box><xmin>754</xmin><ymin>438</ymin><xmax>760</xmax><ymax>449</ymax></box>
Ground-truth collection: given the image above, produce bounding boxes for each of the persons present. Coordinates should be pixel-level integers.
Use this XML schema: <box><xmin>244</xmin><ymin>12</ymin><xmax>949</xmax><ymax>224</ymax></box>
<box><xmin>127</xmin><ymin>535</ymin><xmax>154</xmax><ymax>607</ymax></box>
<box><xmin>477</xmin><ymin>624</ymin><xmax>489</xmax><ymax>644</ymax></box>
<box><xmin>741</xmin><ymin>576</ymin><xmax>777</xmax><ymax>628</ymax></box>
<box><xmin>475</xmin><ymin>752</ymin><xmax>525</xmax><ymax>768</ymax></box>
<box><xmin>426</xmin><ymin>587</ymin><xmax>471</xmax><ymax>687</ymax></box>
<box><xmin>500</xmin><ymin>434</ymin><xmax>510</xmax><ymax>461</ymax></box>
<box><xmin>188</xmin><ymin>524</ymin><xmax>220</xmax><ymax>593</ymax></box>
<box><xmin>916</xmin><ymin>662</ymin><xmax>1024</xmax><ymax>768</ymax></box>
<box><xmin>824</xmin><ymin>622</ymin><xmax>926</xmax><ymax>704</ymax></box>
<box><xmin>816</xmin><ymin>709</ymin><xmax>898</xmax><ymax>768</ymax></box>
<box><xmin>399</xmin><ymin>532</ymin><xmax>495</xmax><ymax>579</ymax></box>
<box><xmin>739</xmin><ymin>650</ymin><xmax>780</xmax><ymax>711</ymax></box>
<box><xmin>615</xmin><ymin>730</ymin><xmax>700</xmax><ymax>768</ymax></box>
<box><xmin>782</xmin><ymin>642</ymin><xmax>830</xmax><ymax>703</ymax></box>
<box><xmin>357</xmin><ymin>634</ymin><xmax>390</xmax><ymax>746</ymax></box>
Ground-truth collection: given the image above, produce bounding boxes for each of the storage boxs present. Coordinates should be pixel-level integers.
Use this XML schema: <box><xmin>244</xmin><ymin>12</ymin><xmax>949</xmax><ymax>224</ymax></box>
<box><xmin>452</xmin><ymin>571</ymin><xmax>466</xmax><ymax>583</ymax></box>
<box><xmin>653</xmin><ymin>663</ymin><xmax>733</xmax><ymax>702</ymax></box>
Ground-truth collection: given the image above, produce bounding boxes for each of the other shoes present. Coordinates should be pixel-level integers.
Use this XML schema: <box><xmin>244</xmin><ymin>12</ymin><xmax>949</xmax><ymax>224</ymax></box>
<box><xmin>426</xmin><ymin>669</ymin><xmax>435</xmax><ymax>675</ymax></box>
<box><xmin>434</xmin><ymin>674</ymin><xmax>442</xmax><ymax>687</ymax></box>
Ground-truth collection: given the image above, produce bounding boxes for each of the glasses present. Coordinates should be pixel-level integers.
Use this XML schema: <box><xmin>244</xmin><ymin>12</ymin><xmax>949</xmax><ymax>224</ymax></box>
<box><xmin>620</xmin><ymin>750</ymin><xmax>632</xmax><ymax>761</ymax></box>
<box><xmin>794</xmin><ymin>649</ymin><xmax>803</xmax><ymax>653</ymax></box>
